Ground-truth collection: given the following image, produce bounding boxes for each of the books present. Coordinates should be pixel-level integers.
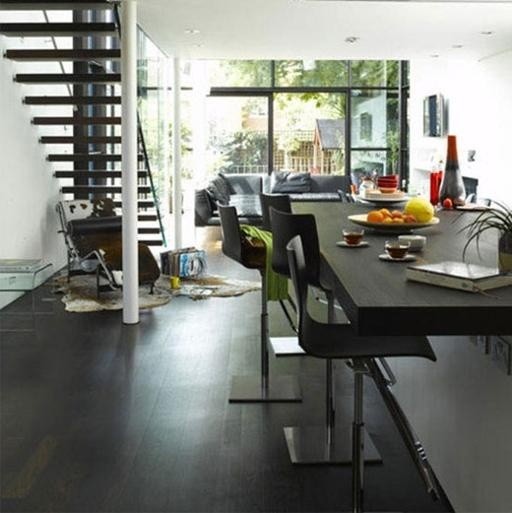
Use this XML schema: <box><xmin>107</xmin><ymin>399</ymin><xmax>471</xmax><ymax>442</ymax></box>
<box><xmin>405</xmin><ymin>261</ymin><xmax>512</xmax><ymax>294</ymax></box>
<box><xmin>0</xmin><ymin>259</ymin><xmax>42</xmax><ymax>271</ymax></box>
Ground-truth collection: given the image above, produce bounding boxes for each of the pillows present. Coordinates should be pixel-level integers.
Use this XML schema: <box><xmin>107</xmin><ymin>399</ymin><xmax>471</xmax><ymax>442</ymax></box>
<box><xmin>270</xmin><ymin>170</ymin><xmax>311</xmax><ymax>193</ymax></box>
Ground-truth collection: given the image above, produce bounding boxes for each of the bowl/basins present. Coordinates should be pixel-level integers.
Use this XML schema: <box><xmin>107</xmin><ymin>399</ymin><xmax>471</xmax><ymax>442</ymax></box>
<box><xmin>378</xmin><ymin>175</ymin><xmax>398</xmax><ymax>192</ymax></box>
<box><xmin>398</xmin><ymin>236</ymin><xmax>426</xmax><ymax>253</ymax></box>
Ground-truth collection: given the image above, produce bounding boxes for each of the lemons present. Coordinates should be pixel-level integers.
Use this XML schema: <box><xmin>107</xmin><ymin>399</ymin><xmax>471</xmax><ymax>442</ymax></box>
<box><xmin>405</xmin><ymin>199</ymin><xmax>435</xmax><ymax>223</ymax></box>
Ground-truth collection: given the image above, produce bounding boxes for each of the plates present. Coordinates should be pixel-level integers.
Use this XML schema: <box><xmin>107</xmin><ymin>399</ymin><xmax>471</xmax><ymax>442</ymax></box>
<box><xmin>336</xmin><ymin>241</ymin><xmax>368</xmax><ymax>247</ymax></box>
<box><xmin>358</xmin><ymin>195</ymin><xmax>410</xmax><ymax>208</ymax></box>
<box><xmin>378</xmin><ymin>253</ymin><xmax>416</xmax><ymax>262</ymax></box>
<box><xmin>349</xmin><ymin>214</ymin><xmax>441</xmax><ymax>234</ymax></box>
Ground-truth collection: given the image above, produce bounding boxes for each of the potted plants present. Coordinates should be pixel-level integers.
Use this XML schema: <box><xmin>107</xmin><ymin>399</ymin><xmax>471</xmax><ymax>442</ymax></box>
<box><xmin>450</xmin><ymin>198</ymin><xmax>511</xmax><ymax>271</ymax></box>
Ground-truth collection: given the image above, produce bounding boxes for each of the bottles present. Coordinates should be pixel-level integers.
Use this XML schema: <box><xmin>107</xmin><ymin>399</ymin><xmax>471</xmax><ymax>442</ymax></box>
<box><xmin>359</xmin><ymin>176</ymin><xmax>376</xmax><ymax>195</ymax></box>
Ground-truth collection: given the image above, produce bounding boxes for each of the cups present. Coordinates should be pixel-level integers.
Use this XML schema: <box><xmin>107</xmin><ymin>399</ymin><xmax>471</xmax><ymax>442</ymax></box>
<box><xmin>430</xmin><ymin>173</ymin><xmax>443</xmax><ymax>204</ymax></box>
<box><xmin>342</xmin><ymin>228</ymin><xmax>365</xmax><ymax>244</ymax></box>
<box><xmin>384</xmin><ymin>240</ymin><xmax>411</xmax><ymax>258</ymax></box>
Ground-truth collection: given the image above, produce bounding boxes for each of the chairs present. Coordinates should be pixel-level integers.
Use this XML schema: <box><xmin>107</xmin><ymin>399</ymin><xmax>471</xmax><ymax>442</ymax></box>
<box><xmin>259</xmin><ymin>191</ymin><xmax>309</xmax><ymax>357</ymax></box>
<box><xmin>55</xmin><ymin>199</ymin><xmax>160</xmax><ymax>299</ymax></box>
<box><xmin>283</xmin><ymin>233</ymin><xmax>441</xmax><ymax>512</ymax></box>
<box><xmin>215</xmin><ymin>200</ymin><xmax>303</xmax><ymax>404</ymax></box>
<box><xmin>268</xmin><ymin>205</ymin><xmax>397</xmax><ymax>466</ymax></box>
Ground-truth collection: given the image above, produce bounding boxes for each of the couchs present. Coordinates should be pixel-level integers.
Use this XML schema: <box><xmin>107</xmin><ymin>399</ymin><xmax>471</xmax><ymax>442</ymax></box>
<box><xmin>195</xmin><ymin>172</ymin><xmax>352</xmax><ymax>228</ymax></box>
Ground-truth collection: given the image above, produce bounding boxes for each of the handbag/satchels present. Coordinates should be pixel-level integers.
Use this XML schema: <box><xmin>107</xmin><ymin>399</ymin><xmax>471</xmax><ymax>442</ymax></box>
<box><xmin>242</xmin><ymin>235</ymin><xmax>266</xmax><ymax>268</ymax></box>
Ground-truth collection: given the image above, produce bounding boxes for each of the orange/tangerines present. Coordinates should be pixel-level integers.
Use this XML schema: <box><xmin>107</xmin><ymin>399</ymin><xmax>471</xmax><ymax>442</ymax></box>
<box><xmin>367</xmin><ymin>208</ymin><xmax>417</xmax><ymax>225</ymax></box>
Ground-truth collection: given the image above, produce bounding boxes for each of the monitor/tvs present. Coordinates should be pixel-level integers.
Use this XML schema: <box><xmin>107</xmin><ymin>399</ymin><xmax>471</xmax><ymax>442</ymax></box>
<box><xmin>423</xmin><ymin>93</ymin><xmax>444</xmax><ymax>137</ymax></box>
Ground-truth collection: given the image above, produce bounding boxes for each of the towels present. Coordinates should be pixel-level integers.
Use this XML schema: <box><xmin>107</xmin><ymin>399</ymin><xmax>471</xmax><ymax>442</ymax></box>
<box><xmin>239</xmin><ymin>223</ymin><xmax>288</xmax><ymax>301</ymax></box>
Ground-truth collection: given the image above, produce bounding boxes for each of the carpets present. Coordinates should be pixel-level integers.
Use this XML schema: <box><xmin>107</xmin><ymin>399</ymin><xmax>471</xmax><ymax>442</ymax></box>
<box><xmin>42</xmin><ymin>260</ymin><xmax>262</xmax><ymax>313</ymax></box>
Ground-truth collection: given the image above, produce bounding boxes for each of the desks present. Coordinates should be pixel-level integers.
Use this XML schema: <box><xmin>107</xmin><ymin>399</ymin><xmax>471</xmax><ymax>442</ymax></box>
<box><xmin>0</xmin><ymin>259</ymin><xmax>56</xmax><ymax>332</ymax></box>
<box><xmin>289</xmin><ymin>202</ymin><xmax>511</xmax><ymax>336</ymax></box>
